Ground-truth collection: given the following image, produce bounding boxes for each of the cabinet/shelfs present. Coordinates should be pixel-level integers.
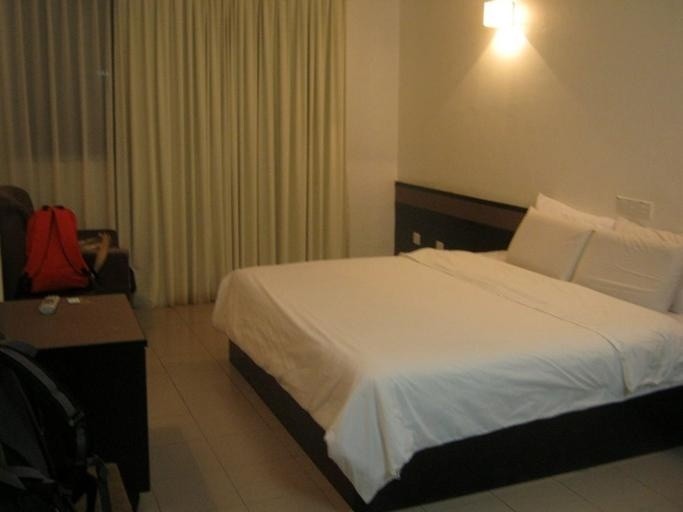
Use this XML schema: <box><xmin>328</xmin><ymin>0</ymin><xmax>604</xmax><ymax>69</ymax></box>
<box><xmin>2</xmin><ymin>286</ymin><xmax>153</xmax><ymax>506</ymax></box>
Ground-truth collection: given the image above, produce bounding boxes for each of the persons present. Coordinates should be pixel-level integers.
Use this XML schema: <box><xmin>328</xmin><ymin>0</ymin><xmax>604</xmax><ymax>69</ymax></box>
<box><xmin>81</xmin><ymin>231</ymin><xmax>111</xmax><ymax>275</ymax></box>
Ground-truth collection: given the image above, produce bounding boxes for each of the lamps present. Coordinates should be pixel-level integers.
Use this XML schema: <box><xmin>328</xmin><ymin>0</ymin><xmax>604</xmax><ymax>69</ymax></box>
<box><xmin>481</xmin><ymin>0</ymin><xmax>516</xmax><ymax>29</ymax></box>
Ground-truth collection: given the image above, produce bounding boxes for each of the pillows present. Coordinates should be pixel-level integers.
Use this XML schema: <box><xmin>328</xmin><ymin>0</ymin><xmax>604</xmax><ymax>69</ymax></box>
<box><xmin>533</xmin><ymin>190</ymin><xmax>614</xmax><ymax>236</ymax></box>
<box><xmin>572</xmin><ymin>227</ymin><xmax>683</xmax><ymax>315</ymax></box>
<box><xmin>610</xmin><ymin>215</ymin><xmax>682</xmax><ymax>246</ymax></box>
<box><xmin>502</xmin><ymin>202</ymin><xmax>592</xmax><ymax>285</ymax></box>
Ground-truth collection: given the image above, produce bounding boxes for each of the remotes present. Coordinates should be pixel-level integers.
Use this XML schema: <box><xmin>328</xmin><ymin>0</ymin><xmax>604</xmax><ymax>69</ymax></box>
<box><xmin>37</xmin><ymin>294</ymin><xmax>60</xmax><ymax>315</ymax></box>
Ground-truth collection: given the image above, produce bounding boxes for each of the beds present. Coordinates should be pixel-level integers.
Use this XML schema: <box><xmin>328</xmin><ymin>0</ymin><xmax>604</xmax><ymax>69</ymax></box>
<box><xmin>204</xmin><ymin>187</ymin><xmax>682</xmax><ymax>512</ymax></box>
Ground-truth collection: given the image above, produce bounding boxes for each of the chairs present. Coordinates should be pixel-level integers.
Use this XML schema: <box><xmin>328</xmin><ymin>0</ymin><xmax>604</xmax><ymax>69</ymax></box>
<box><xmin>1</xmin><ymin>182</ymin><xmax>136</xmax><ymax>295</ymax></box>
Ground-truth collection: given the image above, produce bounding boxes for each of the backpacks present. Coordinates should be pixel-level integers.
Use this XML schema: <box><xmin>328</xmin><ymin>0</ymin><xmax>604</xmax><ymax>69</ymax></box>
<box><xmin>0</xmin><ymin>340</ymin><xmax>103</xmax><ymax>512</ymax></box>
<box><xmin>19</xmin><ymin>204</ymin><xmax>90</xmax><ymax>295</ymax></box>
